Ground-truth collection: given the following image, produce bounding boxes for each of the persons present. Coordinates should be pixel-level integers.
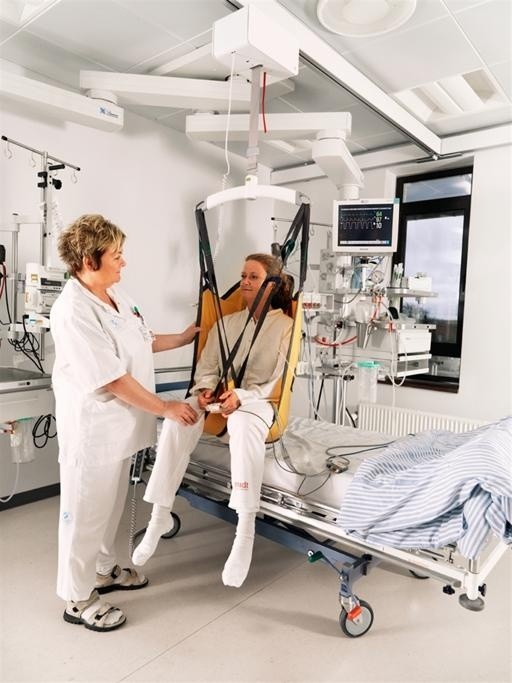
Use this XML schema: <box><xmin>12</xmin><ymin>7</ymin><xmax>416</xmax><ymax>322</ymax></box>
<box><xmin>131</xmin><ymin>252</ymin><xmax>295</xmax><ymax>587</ymax></box>
<box><xmin>49</xmin><ymin>216</ymin><xmax>204</xmax><ymax>633</ymax></box>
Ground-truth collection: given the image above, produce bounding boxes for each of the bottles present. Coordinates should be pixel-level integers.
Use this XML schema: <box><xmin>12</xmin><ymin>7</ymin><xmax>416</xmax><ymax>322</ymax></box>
<box><xmin>11</xmin><ymin>417</ymin><xmax>36</xmax><ymax>465</ymax></box>
<box><xmin>357</xmin><ymin>360</ymin><xmax>381</xmax><ymax>404</ymax></box>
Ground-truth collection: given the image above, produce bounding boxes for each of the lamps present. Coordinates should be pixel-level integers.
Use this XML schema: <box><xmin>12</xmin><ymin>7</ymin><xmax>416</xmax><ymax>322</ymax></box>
<box><xmin>315</xmin><ymin>0</ymin><xmax>418</xmax><ymax>39</ymax></box>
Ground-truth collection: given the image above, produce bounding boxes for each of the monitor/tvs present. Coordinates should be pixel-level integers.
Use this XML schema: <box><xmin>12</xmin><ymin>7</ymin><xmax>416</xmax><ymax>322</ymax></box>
<box><xmin>332</xmin><ymin>199</ymin><xmax>401</xmax><ymax>253</ymax></box>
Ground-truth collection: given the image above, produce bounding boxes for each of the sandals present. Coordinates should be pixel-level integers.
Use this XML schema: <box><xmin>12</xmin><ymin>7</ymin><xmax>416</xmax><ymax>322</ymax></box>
<box><xmin>95</xmin><ymin>565</ymin><xmax>149</xmax><ymax>596</ymax></box>
<box><xmin>63</xmin><ymin>590</ymin><xmax>127</xmax><ymax>632</ymax></box>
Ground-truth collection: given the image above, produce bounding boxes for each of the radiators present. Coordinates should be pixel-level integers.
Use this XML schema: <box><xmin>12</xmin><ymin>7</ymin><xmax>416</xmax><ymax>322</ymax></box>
<box><xmin>356</xmin><ymin>402</ymin><xmax>482</xmax><ymax>437</ymax></box>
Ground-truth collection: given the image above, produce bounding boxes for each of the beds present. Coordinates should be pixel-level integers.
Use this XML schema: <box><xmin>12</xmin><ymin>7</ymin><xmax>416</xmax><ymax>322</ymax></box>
<box><xmin>144</xmin><ymin>416</ymin><xmax>512</xmax><ymax>638</ymax></box>
